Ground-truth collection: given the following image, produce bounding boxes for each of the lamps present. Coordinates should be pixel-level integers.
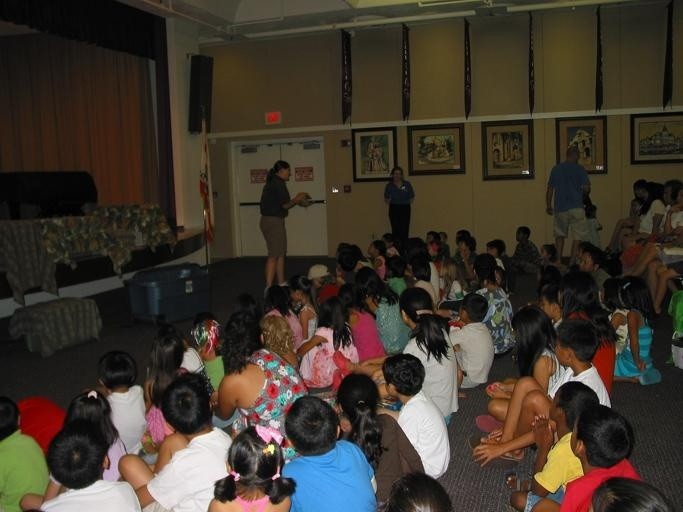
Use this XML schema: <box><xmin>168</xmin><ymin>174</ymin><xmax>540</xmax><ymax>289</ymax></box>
<box><xmin>475</xmin><ymin>0</ymin><xmax>513</xmax><ymax>17</ymax></box>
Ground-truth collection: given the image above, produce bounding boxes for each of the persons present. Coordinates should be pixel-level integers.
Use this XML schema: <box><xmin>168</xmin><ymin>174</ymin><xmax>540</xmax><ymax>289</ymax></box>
<box><xmin>207</xmin><ymin>425</ymin><xmax>296</xmax><ymax>512</ymax></box>
<box><xmin>118</xmin><ymin>372</ymin><xmax>232</xmax><ymax>512</ymax></box>
<box><xmin>387</xmin><ymin>472</ymin><xmax>454</xmax><ymax>512</ymax></box>
<box><xmin>528</xmin><ymin>404</ymin><xmax>641</xmax><ymax>512</ymax></box>
<box><xmin>281</xmin><ymin>395</ymin><xmax>378</xmax><ymax>512</ymax></box>
<box><xmin>0</xmin><ymin>397</ymin><xmax>50</xmax><ymax>512</ymax></box>
<box><xmin>41</xmin><ymin>420</ymin><xmax>142</xmax><ymax>512</ymax></box>
<box><xmin>384</xmin><ymin>166</ymin><xmax>414</xmax><ymax>239</ymax></box>
<box><xmin>545</xmin><ymin>146</ymin><xmax>590</xmax><ymax>267</ymax></box>
<box><xmin>588</xmin><ymin>476</ymin><xmax>671</xmax><ymax>511</ymax></box>
<box><xmin>97</xmin><ymin>229</ymin><xmax>659</xmax><ymax>477</ymax></box>
<box><xmin>605</xmin><ymin>180</ymin><xmax>681</xmax><ymax>313</ymax></box>
<box><xmin>258</xmin><ymin>159</ymin><xmax>309</xmax><ymax>296</ymax></box>
<box><xmin>21</xmin><ymin>390</ymin><xmax>129</xmax><ymax>511</ymax></box>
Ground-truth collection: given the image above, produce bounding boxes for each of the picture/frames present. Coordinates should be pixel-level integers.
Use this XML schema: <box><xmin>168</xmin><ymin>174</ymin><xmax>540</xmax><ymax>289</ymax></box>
<box><xmin>630</xmin><ymin>111</ymin><xmax>683</xmax><ymax>166</ymax></box>
<box><xmin>480</xmin><ymin>119</ymin><xmax>535</xmax><ymax>181</ymax></box>
<box><xmin>555</xmin><ymin>115</ymin><xmax>608</xmax><ymax>175</ymax></box>
<box><xmin>406</xmin><ymin>123</ymin><xmax>465</xmax><ymax>175</ymax></box>
<box><xmin>351</xmin><ymin>127</ymin><xmax>398</xmax><ymax>183</ymax></box>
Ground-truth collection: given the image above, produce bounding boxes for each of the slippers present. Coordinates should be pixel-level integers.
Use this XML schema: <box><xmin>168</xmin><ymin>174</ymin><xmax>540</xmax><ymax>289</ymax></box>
<box><xmin>477</xmin><ymin>416</ymin><xmax>525</xmax><ymax>461</ymax></box>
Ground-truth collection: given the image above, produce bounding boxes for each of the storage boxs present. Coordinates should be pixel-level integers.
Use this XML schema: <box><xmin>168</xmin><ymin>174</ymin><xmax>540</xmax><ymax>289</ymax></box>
<box><xmin>122</xmin><ymin>262</ymin><xmax>209</xmax><ymax>337</ymax></box>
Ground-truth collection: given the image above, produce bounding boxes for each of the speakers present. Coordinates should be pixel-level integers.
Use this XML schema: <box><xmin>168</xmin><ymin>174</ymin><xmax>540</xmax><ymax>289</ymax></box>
<box><xmin>189</xmin><ymin>54</ymin><xmax>213</xmax><ymax>133</ymax></box>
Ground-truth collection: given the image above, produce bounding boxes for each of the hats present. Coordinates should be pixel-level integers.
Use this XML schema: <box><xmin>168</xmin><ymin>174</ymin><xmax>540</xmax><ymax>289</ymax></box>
<box><xmin>308</xmin><ymin>265</ymin><xmax>330</xmax><ymax>280</ymax></box>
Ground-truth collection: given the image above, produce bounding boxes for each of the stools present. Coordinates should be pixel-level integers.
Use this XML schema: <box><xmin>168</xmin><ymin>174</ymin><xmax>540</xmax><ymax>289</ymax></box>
<box><xmin>9</xmin><ymin>296</ymin><xmax>112</xmax><ymax>356</ymax></box>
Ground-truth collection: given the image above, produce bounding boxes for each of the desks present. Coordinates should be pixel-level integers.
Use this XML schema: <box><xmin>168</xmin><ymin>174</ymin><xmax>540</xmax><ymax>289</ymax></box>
<box><xmin>0</xmin><ymin>214</ymin><xmax>97</xmax><ymax>268</ymax></box>
<box><xmin>84</xmin><ymin>203</ymin><xmax>177</xmax><ymax>257</ymax></box>
